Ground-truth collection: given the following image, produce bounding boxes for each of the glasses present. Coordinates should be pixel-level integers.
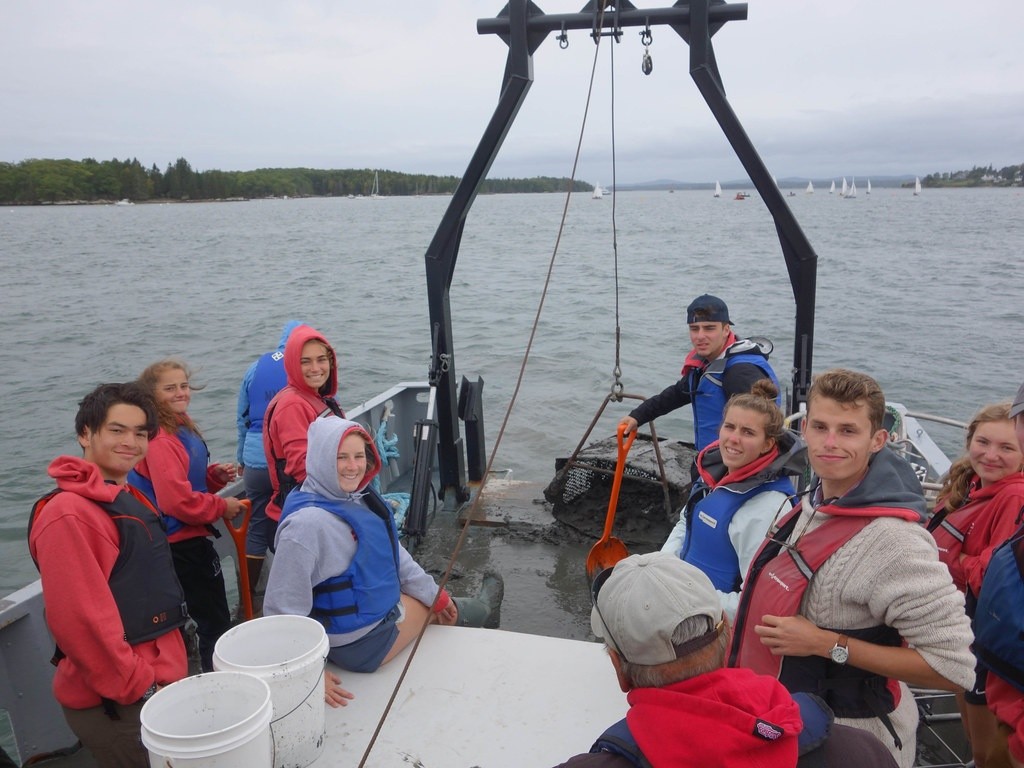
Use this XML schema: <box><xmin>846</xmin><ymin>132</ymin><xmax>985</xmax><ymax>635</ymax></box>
<box><xmin>591</xmin><ymin>567</ymin><xmax>628</xmax><ymax>663</ymax></box>
<box><xmin>764</xmin><ymin>489</ymin><xmax>840</xmax><ymax>553</ymax></box>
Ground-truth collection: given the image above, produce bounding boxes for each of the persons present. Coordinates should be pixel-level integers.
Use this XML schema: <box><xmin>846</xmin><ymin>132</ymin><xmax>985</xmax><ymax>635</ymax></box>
<box><xmin>658</xmin><ymin>378</ymin><xmax>799</xmax><ymax>636</ymax></box>
<box><xmin>547</xmin><ymin>551</ymin><xmax>896</xmax><ymax>768</ymax></box>
<box><xmin>125</xmin><ymin>362</ymin><xmax>248</xmax><ymax>679</ymax></box>
<box><xmin>722</xmin><ymin>369</ymin><xmax>977</xmax><ymax>768</ymax></box>
<box><xmin>264</xmin><ymin>325</ymin><xmax>346</xmax><ymax>555</ymax></box>
<box><xmin>619</xmin><ymin>294</ymin><xmax>782</xmax><ymax>485</ymax></box>
<box><xmin>29</xmin><ymin>382</ymin><xmax>189</xmax><ymax>768</ymax></box>
<box><xmin>971</xmin><ymin>384</ymin><xmax>1024</xmax><ymax>768</ymax></box>
<box><xmin>925</xmin><ymin>403</ymin><xmax>1023</xmax><ymax>593</ymax></box>
<box><xmin>236</xmin><ymin>322</ymin><xmax>300</xmax><ymax>599</ymax></box>
<box><xmin>261</xmin><ymin>416</ymin><xmax>504</xmax><ymax>709</ymax></box>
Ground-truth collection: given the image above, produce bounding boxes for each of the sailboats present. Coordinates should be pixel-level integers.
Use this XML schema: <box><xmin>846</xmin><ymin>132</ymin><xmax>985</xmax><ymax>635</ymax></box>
<box><xmin>713</xmin><ymin>177</ymin><xmax>922</xmax><ymax>199</ymax></box>
<box><xmin>592</xmin><ymin>182</ymin><xmax>611</xmax><ymax>199</ymax></box>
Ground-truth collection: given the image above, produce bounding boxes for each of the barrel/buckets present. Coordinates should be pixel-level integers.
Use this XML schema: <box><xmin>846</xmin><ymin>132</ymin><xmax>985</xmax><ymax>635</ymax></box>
<box><xmin>212</xmin><ymin>614</ymin><xmax>330</xmax><ymax>768</ymax></box>
<box><xmin>140</xmin><ymin>669</ymin><xmax>275</xmax><ymax>768</ymax></box>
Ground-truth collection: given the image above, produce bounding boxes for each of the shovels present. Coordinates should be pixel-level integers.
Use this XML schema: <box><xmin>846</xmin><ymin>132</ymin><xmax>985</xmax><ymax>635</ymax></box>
<box><xmin>585</xmin><ymin>423</ymin><xmax>637</xmax><ymax>591</ymax></box>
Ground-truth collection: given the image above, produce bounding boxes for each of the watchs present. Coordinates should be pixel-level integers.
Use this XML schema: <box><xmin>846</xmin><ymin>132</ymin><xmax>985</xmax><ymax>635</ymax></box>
<box><xmin>829</xmin><ymin>633</ymin><xmax>850</xmax><ymax>666</ymax></box>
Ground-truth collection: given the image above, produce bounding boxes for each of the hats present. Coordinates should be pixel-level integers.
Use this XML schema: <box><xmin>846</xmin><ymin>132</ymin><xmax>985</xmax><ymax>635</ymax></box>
<box><xmin>687</xmin><ymin>294</ymin><xmax>734</xmax><ymax>325</ymax></box>
<box><xmin>590</xmin><ymin>551</ymin><xmax>724</xmax><ymax>665</ymax></box>
<box><xmin>1008</xmin><ymin>382</ymin><xmax>1024</xmax><ymax>419</ymax></box>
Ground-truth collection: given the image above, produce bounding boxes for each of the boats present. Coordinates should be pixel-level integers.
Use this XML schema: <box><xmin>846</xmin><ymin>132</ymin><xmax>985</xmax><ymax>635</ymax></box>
<box><xmin>0</xmin><ymin>2</ymin><xmax>1024</xmax><ymax>767</ymax></box>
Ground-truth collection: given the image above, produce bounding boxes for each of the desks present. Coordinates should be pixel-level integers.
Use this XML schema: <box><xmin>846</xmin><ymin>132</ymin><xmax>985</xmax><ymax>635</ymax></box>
<box><xmin>305</xmin><ymin>625</ymin><xmax>632</xmax><ymax>768</ymax></box>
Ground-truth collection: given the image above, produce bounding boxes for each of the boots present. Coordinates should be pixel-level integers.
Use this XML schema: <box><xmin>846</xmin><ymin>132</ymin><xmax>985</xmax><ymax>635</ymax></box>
<box><xmin>450</xmin><ymin>567</ymin><xmax>504</xmax><ymax>629</ymax></box>
